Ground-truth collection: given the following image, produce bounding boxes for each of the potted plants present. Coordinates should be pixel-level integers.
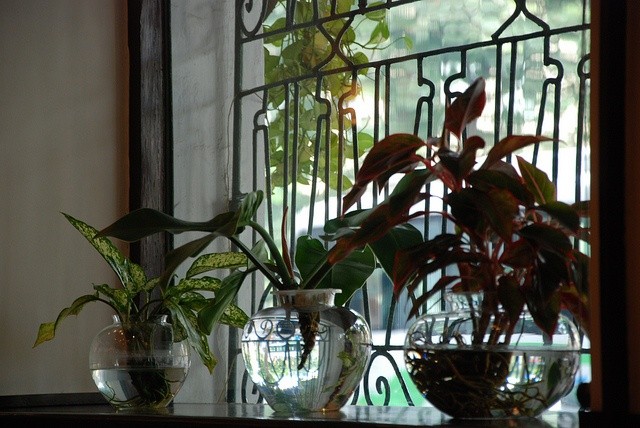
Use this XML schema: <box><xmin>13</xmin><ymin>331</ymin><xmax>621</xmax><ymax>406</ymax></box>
<box><xmin>91</xmin><ymin>191</ymin><xmax>423</xmax><ymax>416</ymax></box>
<box><xmin>31</xmin><ymin>211</ymin><xmax>250</xmax><ymax>413</ymax></box>
<box><xmin>326</xmin><ymin>77</ymin><xmax>592</xmax><ymax>422</ymax></box>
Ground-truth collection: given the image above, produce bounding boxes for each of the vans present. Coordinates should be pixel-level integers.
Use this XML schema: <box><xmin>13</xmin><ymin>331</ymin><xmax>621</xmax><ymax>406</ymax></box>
<box><xmin>270</xmin><ymin>147</ymin><xmax>591</xmax><ymax>405</ymax></box>
